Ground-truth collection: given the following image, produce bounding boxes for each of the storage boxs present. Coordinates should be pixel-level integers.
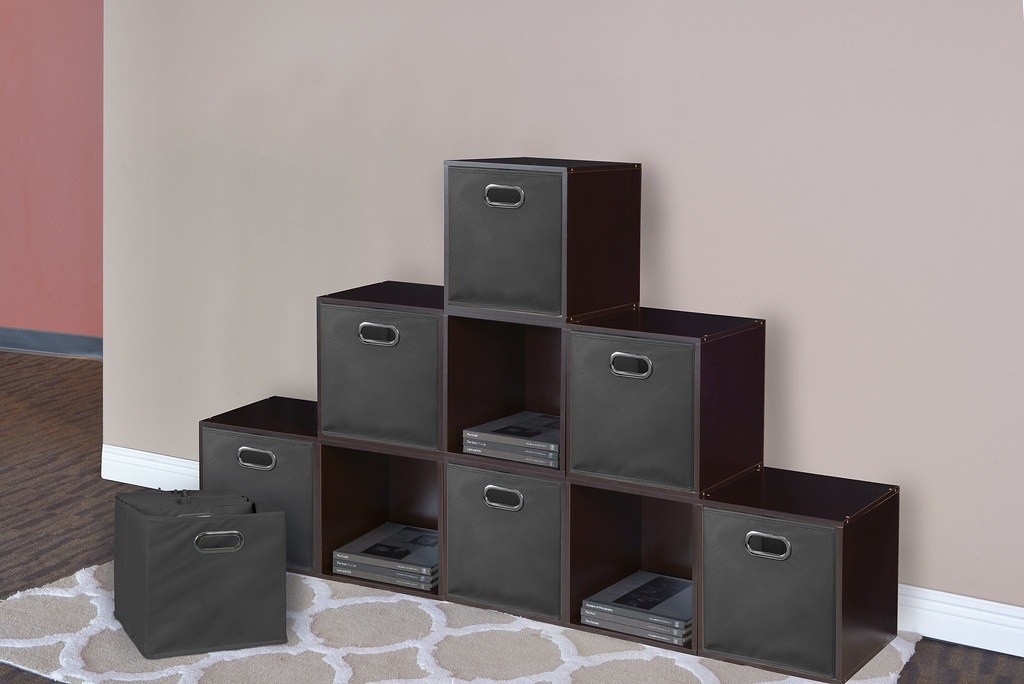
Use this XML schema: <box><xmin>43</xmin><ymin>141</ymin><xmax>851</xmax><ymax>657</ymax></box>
<box><xmin>112</xmin><ymin>154</ymin><xmax>901</xmax><ymax>684</ymax></box>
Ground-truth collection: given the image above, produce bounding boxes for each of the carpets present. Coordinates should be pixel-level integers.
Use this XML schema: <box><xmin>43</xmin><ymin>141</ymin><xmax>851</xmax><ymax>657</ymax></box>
<box><xmin>0</xmin><ymin>556</ymin><xmax>923</xmax><ymax>684</ymax></box>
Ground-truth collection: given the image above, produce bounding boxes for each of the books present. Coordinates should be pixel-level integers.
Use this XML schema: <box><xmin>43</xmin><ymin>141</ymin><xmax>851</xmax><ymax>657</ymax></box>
<box><xmin>462</xmin><ymin>410</ymin><xmax>560</xmax><ymax>470</ymax></box>
<box><xmin>581</xmin><ymin>569</ymin><xmax>693</xmax><ymax>646</ymax></box>
<box><xmin>332</xmin><ymin>521</ymin><xmax>438</xmax><ymax>592</ymax></box>
